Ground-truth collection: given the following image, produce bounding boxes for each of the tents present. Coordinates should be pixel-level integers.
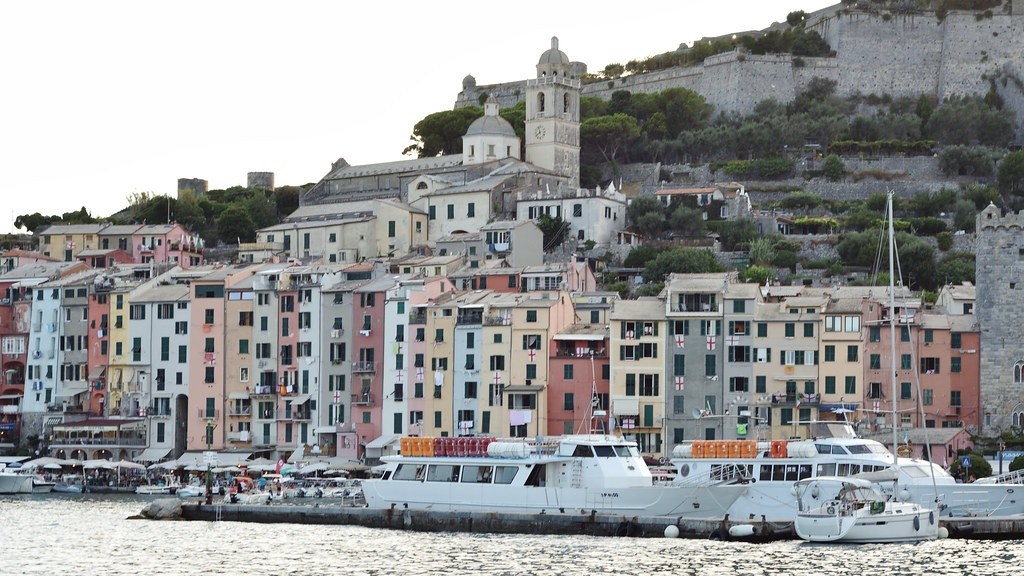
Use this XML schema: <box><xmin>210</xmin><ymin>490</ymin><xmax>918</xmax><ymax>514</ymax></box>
<box><xmin>240</xmin><ymin>456</ymin><xmax>392</xmax><ymax>478</ymax></box>
<box><xmin>0</xmin><ymin>457</ymin><xmax>182</xmax><ymax>483</ymax></box>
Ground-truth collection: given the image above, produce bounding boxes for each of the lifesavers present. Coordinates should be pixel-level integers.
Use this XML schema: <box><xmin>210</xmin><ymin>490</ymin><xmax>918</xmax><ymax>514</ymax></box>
<box><xmin>690</xmin><ymin>440</ymin><xmax>758</xmax><ymax>458</ymax></box>
<box><xmin>771</xmin><ymin>440</ymin><xmax>786</xmax><ymax>458</ymax></box>
<box><xmin>400</xmin><ymin>436</ymin><xmax>497</xmax><ymax>457</ymax></box>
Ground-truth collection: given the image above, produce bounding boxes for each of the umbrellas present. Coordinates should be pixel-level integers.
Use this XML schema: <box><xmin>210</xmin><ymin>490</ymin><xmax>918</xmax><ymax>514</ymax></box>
<box><xmin>44</xmin><ymin>463</ymin><xmax>62</xmax><ymax>477</ymax></box>
<box><xmin>183</xmin><ymin>465</ymin><xmax>242</xmax><ymax>478</ymax></box>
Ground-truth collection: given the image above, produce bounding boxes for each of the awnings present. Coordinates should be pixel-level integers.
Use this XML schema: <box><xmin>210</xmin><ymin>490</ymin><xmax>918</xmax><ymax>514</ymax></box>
<box><xmin>0</xmin><ymin>456</ymin><xmax>32</xmax><ymax>463</ymax></box>
<box><xmin>132</xmin><ymin>448</ymin><xmax>172</xmax><ymax>462</ymax></box>
<box><xmin>176</xmin><ymin>452</ymin><xmax>253</xmax><ymax>467</ymax></box>
<box><xmin>820</xmin><ymin>404</ymin><xmax>858</xmax><ymax>410</ymax></box>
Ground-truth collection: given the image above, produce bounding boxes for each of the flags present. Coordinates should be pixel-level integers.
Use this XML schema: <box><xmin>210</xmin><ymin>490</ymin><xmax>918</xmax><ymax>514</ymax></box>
<box><xmin>795</xmin><ymin>384</ymin><xmax>802</xmax><ymax>411</ymax></box>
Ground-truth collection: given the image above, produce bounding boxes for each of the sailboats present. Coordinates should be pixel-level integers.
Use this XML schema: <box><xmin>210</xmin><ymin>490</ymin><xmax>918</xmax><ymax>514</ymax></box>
<box><xmin>793</xmin><ymin>185</ymin><xmax>942</xmax><ymax>542</ymax></box>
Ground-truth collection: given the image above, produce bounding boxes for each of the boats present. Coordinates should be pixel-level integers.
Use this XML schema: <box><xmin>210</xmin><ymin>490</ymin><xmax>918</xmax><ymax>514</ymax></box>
<box><xmin>359</xmin><ymin>346</ymin><xmax>1023</xmax><ymax>539</ymax></box>
<box><xmin>135</xmin><ymin>474</ymin><xmax>179</xmax><ymax>494</ymax></box>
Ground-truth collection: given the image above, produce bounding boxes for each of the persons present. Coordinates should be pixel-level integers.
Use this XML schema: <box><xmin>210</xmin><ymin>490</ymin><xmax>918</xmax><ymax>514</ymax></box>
<box><xmin>45</xmin><ymin>471</ymin><xmax>50</xmax><ymax>481</ymax></box>
<box><xmin>56</xmin><ymin>470</ymin><xmax>62</xmax><ymax>479</ymax></box>
<box><xmin>955</xmin><ymin>464</ymin><xmax>976</xmax><ymax>483</ymax></box>
<box><xmin>90</xmin><ymin>468</ymin><xmax>130</xmax><ymax>487</ymax></box>
<box><xmin>240</xmin><ymin>480</ymin><xmax>247</xmax><ymax>494</ymax></box>
<box><xmin>259</xmin><ymin>476</ymin><xmax>266</xmax><ymax>492</ymax></box>
<box><xmin>276</xmin><ymin>480</ymin><xmax>282</xmax><ymax>496</ymax></box>
